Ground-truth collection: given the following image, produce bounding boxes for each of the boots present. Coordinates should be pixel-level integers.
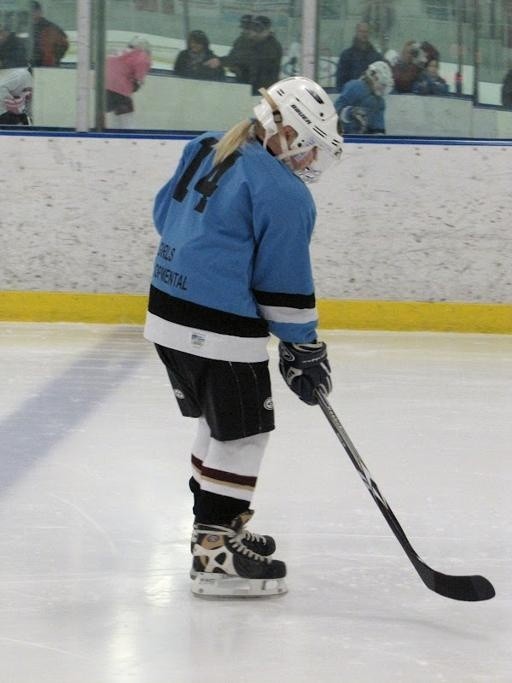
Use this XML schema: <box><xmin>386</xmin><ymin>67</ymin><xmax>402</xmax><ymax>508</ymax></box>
<box><xmin>190</xmin><ymin>505</ymin><xmax>276</xmax><ymax>557</ymax></box>
<box><xmin>191</xmin><ymin>508</ymin><xmax>286</xmax><ymax>580</ymax></box>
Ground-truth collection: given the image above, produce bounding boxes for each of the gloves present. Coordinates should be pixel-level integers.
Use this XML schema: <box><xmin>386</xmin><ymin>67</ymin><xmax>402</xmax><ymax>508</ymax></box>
<box><xmin>278</xmin><ymin>341</ymin><xmax>332</xmax><ymax>406</ymax></box>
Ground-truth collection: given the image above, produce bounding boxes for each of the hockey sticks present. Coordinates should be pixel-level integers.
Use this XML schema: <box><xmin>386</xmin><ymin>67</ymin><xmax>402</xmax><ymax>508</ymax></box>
<box><xmin>315</xmin><ymin>383</ymin><xmax>495</xmax><ymax>600</ymax></box>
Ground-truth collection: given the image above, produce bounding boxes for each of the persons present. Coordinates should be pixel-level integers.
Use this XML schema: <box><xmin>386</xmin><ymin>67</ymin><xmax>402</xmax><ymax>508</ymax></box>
<box><xmin>104</xmin><ymin>36</ymin><xmax>153</xmax><ymax>129</ymax></box>
<box><xmin>0</xmin><ymin>1</ymin><xmax>69</xmax><ymax>125</ymax></box>
<box><xmin>143</xmin><ymin>75</ymin><xmax>345</xmax><ymax>580</ymax></box>
<box><xmin>174</xmin><ymin>14</ymin><xmax>449</xmax><ymax>135</ymax></box>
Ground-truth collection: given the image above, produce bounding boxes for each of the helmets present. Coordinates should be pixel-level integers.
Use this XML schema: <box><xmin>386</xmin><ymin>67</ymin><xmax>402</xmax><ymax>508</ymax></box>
<box><xmin>127</xmin><ymin>34</ymin><xmax>152</xmax><ymax>55</ymax></box>
<box><xmin>366</xmin><ymin>60</ymin><xmax>394</xmax><ymax>96</ymax></box>
<box><xmin>253</xmin><ymin>75</ymin><xmax>344</xmax><ymax>185</ymax></box>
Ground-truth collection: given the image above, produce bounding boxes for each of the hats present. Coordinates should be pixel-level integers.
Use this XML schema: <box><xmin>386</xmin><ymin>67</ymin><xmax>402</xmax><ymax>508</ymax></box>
<box><xmin>237</xmin><ymin>14</ymin><xmax>252</xmax><ymax>29</ymax></box>
<box><xmin>250</xmin><ymin>15</ymin><xmax>271</xmax><ymax>33</ymax></box>
